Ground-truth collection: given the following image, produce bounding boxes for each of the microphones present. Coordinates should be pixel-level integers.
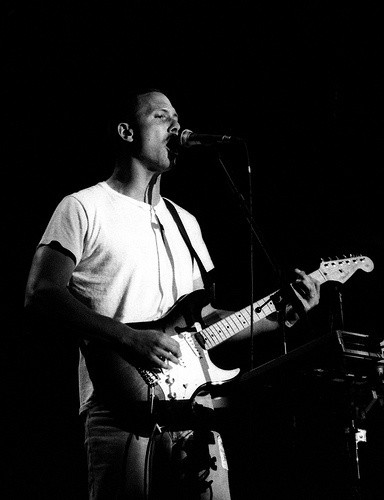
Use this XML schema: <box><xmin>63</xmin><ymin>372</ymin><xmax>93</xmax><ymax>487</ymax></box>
<box><xmin>178</xmin><ymin>129</ymin><xmax>238</xmax><ymax>146</ymax></box>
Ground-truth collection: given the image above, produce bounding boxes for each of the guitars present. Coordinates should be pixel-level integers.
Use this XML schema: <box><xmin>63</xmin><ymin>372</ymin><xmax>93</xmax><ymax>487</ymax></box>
<box><xmin>74</xmin><ymin>252</ymin><xmax>377</xmax><ymax>439</ymax></box>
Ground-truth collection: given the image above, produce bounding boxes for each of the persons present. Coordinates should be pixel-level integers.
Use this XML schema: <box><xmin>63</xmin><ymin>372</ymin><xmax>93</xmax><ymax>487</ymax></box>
<box><xmin>25</xmin><ymin>86</ymin><xmax>321</xmax><ymax>500</ymax></box>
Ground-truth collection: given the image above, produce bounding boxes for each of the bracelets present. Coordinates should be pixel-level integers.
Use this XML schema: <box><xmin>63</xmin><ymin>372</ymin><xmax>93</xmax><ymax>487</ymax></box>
<box><xmin>282</xmin><ymin>305</ymin><xmax>299</xmax><ymax>328</ymax></box>
